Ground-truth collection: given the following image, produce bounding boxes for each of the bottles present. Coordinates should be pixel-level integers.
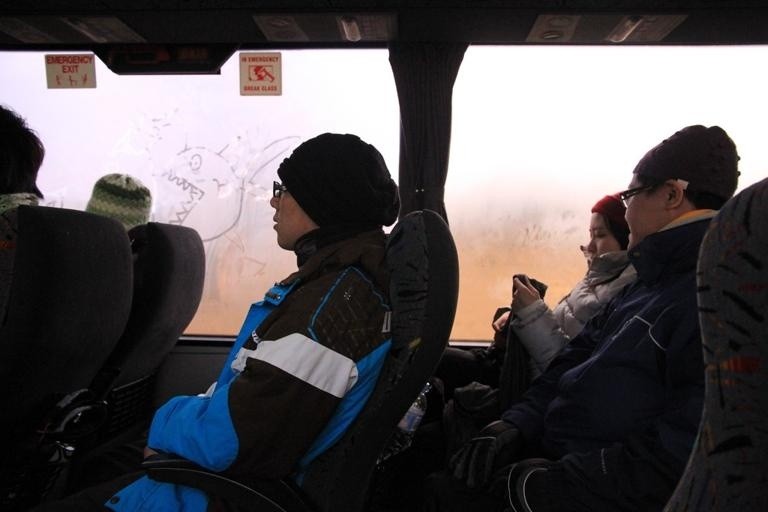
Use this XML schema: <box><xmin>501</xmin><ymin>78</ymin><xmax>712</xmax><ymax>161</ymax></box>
<box><xmin>395</xmin><ymin>384</ymin><xmax>432</xmax><ymax>438</ymax></box>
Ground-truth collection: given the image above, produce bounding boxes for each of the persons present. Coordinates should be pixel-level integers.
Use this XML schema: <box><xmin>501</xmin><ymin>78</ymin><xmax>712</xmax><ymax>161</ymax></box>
<box><xmin>403</xmin><ymin>192</ymin><xmax>638</xmax><ymax>512</ymax></box>
<box><xmin>0</xmin><ymin>104</ymin><xmax>152</xmax><ymax>230</ymax></box>
<box><xmin>403</xmin><ymin>125</ymin><xmax>741</xmax><ymax>511</ymax></box>
<box><xmin>33</xmin><ymin>133</ymin><xmax>399</xmax><ymax>512</ymax></box>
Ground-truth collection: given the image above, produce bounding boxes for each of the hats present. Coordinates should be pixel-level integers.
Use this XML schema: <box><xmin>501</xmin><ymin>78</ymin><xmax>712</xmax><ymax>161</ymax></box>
<box><xmin>591</xmin><ymin>191</ymin><xmax>629</xmax><ymax>229</ymax></box>
<box><xmin>640</xmin><ymin>124</ymin><xmax>739</xmax><ymax>200</ymax></box>
<box><xmin>276</xmin><ymin>132</ymin><xmax>400</xmax><ymax>231</ymax></box>
<box><xmin>84</xmin><ymin>172</ymin><xmax>152</xmax><ymax>232</ymax></box>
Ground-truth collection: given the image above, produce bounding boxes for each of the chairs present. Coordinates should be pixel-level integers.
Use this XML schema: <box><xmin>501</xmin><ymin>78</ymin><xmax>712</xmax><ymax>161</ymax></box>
<box><xmin>615</xmin><ymin>181</ymin><xmax>764</xmax><ymax>507</ymax></box>
<box><xmin>136</xmin><ymin>203</ymin><xmax>466</xmax><ymax>508</ymax></box>
<box><xmin>3</xmin><ymin>200</ymin><xmax>210</xmax><ymax>508</ymax></box>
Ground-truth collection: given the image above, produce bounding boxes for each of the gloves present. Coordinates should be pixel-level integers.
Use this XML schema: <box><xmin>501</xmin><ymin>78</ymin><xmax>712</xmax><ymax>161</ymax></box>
<box><xmin>446</xmin><ymin>419</ymin><xmax>520</xmax><ymax>489</ymax></box>
<box><xmin>489</xmin><ymin>458</ymin><xmax>575</xmax><ymax>500</ymax></box>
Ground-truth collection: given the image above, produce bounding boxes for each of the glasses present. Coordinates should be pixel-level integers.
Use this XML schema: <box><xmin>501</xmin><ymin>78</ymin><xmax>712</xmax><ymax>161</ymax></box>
<box><xmin>619</xmin><ymin>184</ymin><xmax>655</xmax><ymax>207</ymax></box>
<box><xmin>273</xmin><ymin>181</ymin><xmax>288</xmax><ymax>197</ymax></box>
